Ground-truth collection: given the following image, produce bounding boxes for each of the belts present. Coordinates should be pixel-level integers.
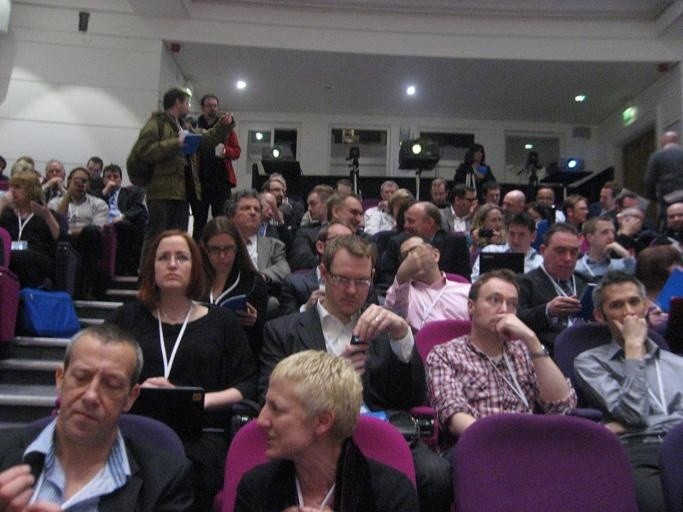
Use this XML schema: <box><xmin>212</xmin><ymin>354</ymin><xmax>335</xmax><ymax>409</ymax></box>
<box><xmin>620</xmin><ymin>434</ymin><xmax>664</xmax><ymax>444</ymax></box>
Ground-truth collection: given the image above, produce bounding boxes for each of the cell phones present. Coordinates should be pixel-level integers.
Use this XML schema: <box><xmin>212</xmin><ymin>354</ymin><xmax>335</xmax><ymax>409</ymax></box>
<box><xmin>350</xmin><ymin>335</ymin><xmax>366</xmax><ymax>355</ymax></box>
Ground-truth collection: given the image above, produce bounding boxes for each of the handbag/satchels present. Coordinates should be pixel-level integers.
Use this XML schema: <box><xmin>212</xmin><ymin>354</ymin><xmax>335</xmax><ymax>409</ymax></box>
<box><xmin>126</xmin><ymin>116</ymin><xmax>164</xmax><ymax>186</ymax></box>
<box><xmin>20</xmin><ymin>287</ymin><xmax>81</xmax><ymax>337</ymax></box>
<box><xmin>386</xmin><ymin>408</ymin><xmax>421</xmax><ymax>447</ymax></box>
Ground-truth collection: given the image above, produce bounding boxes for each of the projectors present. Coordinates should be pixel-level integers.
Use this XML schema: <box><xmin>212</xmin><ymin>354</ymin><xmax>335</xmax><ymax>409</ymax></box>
<box><xmin>261</xmin><ymin>144</ymin><xmax>291</xmax><ymax>161</ymax></box>
<box><xmin>557</xmin><ymin>157</ymin><xmax>585</xmax><ymax>170</ymax></box>
<box><xmin>399</xmin><ymin>139</ymin><xmax>440</xmax><ymax>169</ymax></box>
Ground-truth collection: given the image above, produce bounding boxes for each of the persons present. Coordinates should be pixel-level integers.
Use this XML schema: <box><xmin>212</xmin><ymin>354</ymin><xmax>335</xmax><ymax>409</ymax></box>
<box><xmin>0</xmin><ymin>324</ymin><xmax>196</xmax><ymax>512</ymax></box>
<box><xmin>135</xmin><ymin>88</ymin><xmax>236</xmax><ymax>286</ymax></box>
<box><xmin>200</xmin><ymin>172</ymin><xmax>683</xmax><ymax>338</ymax></box>
<box><xmin>454</xmin><ymin>144</ymin><xmax>496</xmax><ymax>183</ymax></box>
<box><xmin>0</xmin><ymin>154</ymin><xmax>147</xmax><ymax>289</ymax></box>
<box><xmin>424</xmin><ymin>272</ymin><xmax>578</xmax><ymax>460</ymax></box>
<box><xmin>574</xmin><ymin>269</ymin><xmax>683</xmax><ymax>512</ymax></box>
<box><xmin>260</xmin><ymin>234</ymin><xmax>452</xmax><ymax>512</ymax></box>
<box><xmin>232</xmin><ymin>349</ymin><xmax>421</xmax><ymax>512</ymax></box>
<box><xmin>103</xmin><ymin>229</ymin><xmax>260</xmax><ymax>512</ymax></box>
<box><xmin>644</xmin><ymin>131</ymin><xmax>683</xmax><ymax>200</ymax></box>
<box><xmin>187</xmin><ymin>96</ymin><xmax>242</xmax><ymax>236</ymax></box>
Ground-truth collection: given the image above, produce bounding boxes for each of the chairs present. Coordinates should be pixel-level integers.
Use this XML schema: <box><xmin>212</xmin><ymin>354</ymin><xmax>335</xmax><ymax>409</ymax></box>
<box><xmin>656</xmin><ymin>421</ymin><xmax>683</xmax><ymax>512</ymax></box>
<box><xmin>549</xmin><ymin>319</ymin><xmax>668</xmax><ymax>422</ymax></box>
<box><xmin>0</xmin><ymin>174</ymin><xmax>151</xmax><ymax>341</ymax></box>
<box><xmin>450</xmin><ymin>412</ymin><xmax>638</xmax><ymax>509</ymax></box>
<box><xmin>359</xmin><ymin>197</ymin><xmax>378</xmax><ymax>214</ymax></box>
<box><xmin>222</xmin><ymin>411</ymin><xmax>422</xmax><ymax>512</ymax></box>
<box><xmin>410</xmin><ymin>317</ymin><xmax>471</xmax><ymax>456</ymax></box>
<box><xmin>443</xmin><ymin>271</ymin><xmax>468</xmax><ymax>288</ymax></box>
<box><xmin>576</xmin><ymin>232</ymin><xmax>589</xmax><ymax>255</ymax></box>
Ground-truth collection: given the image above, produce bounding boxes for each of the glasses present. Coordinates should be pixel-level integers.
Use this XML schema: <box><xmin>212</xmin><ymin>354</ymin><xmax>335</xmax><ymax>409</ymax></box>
<box><xmin>208</xmin><ymin>246</ymin><xmax>233</xmax><ymax>254</ymax></box>
<box><xmin>330</xmin><ymin>271</ymin><xmax>371</xmax><ymax>288</ymax></box>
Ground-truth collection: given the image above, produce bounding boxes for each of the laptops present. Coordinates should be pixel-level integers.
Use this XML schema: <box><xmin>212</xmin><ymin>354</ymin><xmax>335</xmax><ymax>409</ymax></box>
<box><xmin>129</xmin><ymin>387</ymin><xmax>207</xmax><ymax>429</ymax></box>
<box><xmin>479</xmin><ymin>252</ymin><xmax>525</xmax><ymax>275</ymax></box>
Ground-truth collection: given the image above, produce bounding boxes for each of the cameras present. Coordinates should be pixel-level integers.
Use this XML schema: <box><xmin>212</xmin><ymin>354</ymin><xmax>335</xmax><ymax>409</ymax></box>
<box><xmin>479</xmin><ymin>228</ymin><xmax>492</xmax><ymax>237</ymax></box>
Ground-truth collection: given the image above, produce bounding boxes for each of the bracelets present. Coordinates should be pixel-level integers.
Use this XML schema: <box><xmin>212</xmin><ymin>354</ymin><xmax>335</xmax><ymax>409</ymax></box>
<box><xmin>529</xmin><ymin>344</ymin><xmax>550</xmax><ymax>359</ymax></box>
